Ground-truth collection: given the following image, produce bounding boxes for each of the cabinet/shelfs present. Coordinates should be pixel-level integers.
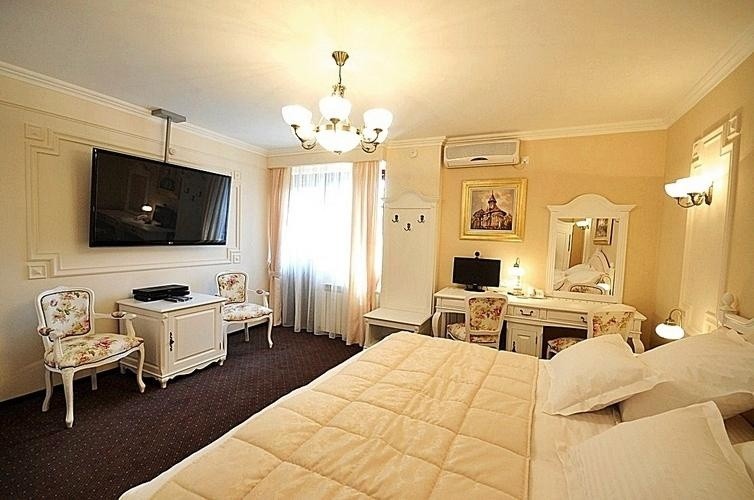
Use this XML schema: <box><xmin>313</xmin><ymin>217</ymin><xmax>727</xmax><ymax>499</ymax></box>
<box><xmin>115</xmin><ymin>292</ymin><xmax>228</xmax><ymax>390</ymax></box>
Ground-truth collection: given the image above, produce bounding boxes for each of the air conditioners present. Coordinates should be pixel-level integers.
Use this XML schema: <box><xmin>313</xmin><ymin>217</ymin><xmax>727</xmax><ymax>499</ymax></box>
<box><xmin>443</xmin><ymin>138</ymin><xmax>520</xmax><ymax>169</ymax></box>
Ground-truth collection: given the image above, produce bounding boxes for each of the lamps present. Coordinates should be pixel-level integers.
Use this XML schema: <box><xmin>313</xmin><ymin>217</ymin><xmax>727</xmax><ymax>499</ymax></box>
<box><xmin>281</xmin><ymin>49</ymin><xmax>393</xmax><ymax>156</ymax></box>
<box><xmin>508</xmin><ymin>257</ymin><xmax>525</xmax><ymax>297</ymax></box>
<box><xmin>575</xmin><ymin>221</ymin><xmax>590</xmax><ymax>230</ymax></box>
<box><xmin>665</xmin><ymin>175</ymin><xmax>714</xmax><ymax>209</ymax></box>
<box><xmin>597</xmin><ymin>276</ymin><xmax>611</xmax><ymax>292</ymax></box>
<box><xmin>655</xmin><ymin>308</ymin><xmax>685</xmax><ymax>339</ymax></box>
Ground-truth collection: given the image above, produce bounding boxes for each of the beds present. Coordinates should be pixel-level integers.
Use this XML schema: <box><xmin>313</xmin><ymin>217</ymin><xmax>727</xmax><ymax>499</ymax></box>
<box><xmin>119</xmin><ymin>292</ymin><xmax>754</xmax><ymax>500</ymax></box>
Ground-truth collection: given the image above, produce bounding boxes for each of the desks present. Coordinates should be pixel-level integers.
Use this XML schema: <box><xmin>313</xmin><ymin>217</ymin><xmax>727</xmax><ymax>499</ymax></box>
<box><xmin>432</xmin><ymin>287</ymin><xmax>647</xmax><ymax>359</ymax></box>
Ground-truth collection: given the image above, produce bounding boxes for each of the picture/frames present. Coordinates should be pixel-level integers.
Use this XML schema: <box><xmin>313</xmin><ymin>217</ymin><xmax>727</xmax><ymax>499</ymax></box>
<box><xmin>592</xmin><ymin>219</ymin><xmax>613</xmax><ymax>245</ymax></box>
<box><xmin>459</xmin><ymin>178</ymin><xmax>528</xmax><ymax>243</ymax></box>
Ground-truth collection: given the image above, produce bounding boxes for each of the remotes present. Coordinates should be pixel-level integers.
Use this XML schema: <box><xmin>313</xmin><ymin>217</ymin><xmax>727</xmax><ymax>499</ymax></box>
<box><xmin>171</xmin><ymin>296</ymin><xmax>188</xmax><ymax>302</ymax></box>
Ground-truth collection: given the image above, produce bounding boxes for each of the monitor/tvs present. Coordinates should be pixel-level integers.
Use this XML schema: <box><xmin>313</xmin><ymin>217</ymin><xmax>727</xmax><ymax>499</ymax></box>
<box><xmin>151</xmin><ymin>204</ymin><xmax>173</xmax><ymax>229</ymax></box>
<box><xmin>451</xmin><ymin>256</ymin><xmax>501</xmax><ymax>292</ymax></box>
<box><xmin>87</xmin><ymin>147</ymin><xmax>232</xmax><ymax>248</ymax></box>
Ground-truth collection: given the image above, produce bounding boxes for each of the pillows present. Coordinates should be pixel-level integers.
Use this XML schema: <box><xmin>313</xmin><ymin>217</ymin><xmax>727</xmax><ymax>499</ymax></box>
<box><xmin>554</xmin><ymin>263</ymin><xmax>604</xmax><ymax>291</ymax></box>
<box><xmin>544</xmin><ymin>329</ymin><xmax>754</xmax><ymax>500</ymax></box>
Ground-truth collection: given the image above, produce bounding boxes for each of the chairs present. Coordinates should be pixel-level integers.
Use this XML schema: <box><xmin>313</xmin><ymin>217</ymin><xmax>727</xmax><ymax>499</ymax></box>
<box><xmin>215</xmin><ymin>271</ymin><xmax>274</xmax><ymax>349</ymax></box>
<box><xmin>569</xmin><ymin>283</ymin><xmax>606</xmax><ymax>295</ymax></box>
<box><xmin>546</xmin><ymin>304</ymin><xmax>636</xmax><ymax>360</ymax></box>
<box><xmin>446</xmin><ymin>292</ymin><xmax>508</xmax><ymax>351</ymax></box>
<box><xmin>34</xmin><ymin>285</ymin><xmax>147</xmax><ymax>429</ymax></box>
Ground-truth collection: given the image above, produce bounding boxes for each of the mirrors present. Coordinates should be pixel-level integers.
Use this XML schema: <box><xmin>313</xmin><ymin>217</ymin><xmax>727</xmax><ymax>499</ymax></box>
<box><xmin>545</xmin><ymin>193</ymin><xmax>637</xmax><ymax>303</ymax></box>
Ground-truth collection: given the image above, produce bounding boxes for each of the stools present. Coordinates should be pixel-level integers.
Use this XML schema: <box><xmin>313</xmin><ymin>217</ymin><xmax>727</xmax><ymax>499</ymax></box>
<box><xmin>362</xmin><ymin>307</ymin><xmax>433</xmax><ymax>351</ymax></box>
